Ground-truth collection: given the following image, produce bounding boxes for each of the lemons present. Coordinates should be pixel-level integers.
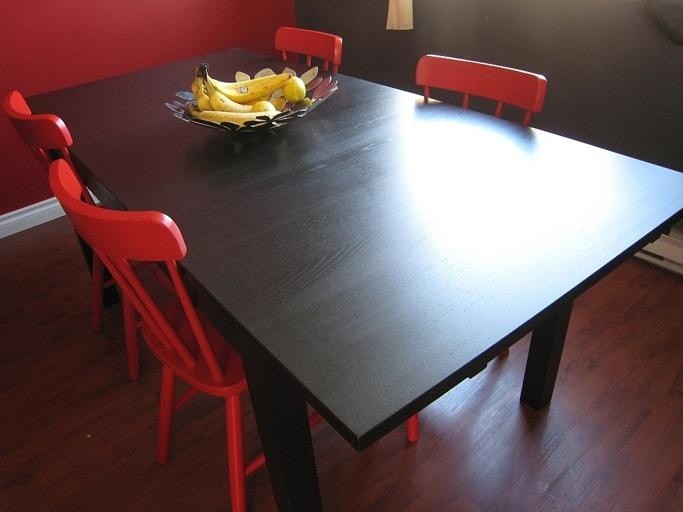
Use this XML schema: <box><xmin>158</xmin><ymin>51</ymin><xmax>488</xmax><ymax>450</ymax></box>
<box><xmin>282</xmin><ymin>75</ymin><xmax>307</xmax><ymax>104</ymax></box>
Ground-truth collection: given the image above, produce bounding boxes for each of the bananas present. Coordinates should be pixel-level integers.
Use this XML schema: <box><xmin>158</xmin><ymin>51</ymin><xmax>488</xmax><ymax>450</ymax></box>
<box><xmin>186</xmin><ymin>61</ymin><xmax>291</xmax><ymax>123</ymax></box>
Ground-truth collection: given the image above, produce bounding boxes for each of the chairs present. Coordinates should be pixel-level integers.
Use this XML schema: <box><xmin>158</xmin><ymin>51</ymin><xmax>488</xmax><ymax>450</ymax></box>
<box><xmin>4</xmin><ymin>91</ymin><xmax>140</xmax><ymax>380</ymax></box>
<box><xmin>275</xmin><ymin>30</ymin><xmax>342</xmax><ymax>74</ymax></box>
<box><xmin>49</xmin><ymin>159</ymin><xmax>419</xmax><ymax>507</ymax></box>
<box><xmin>415</xmin><ymin>54</ymin><xmax>546</xmax><ymax>127</ymax></box>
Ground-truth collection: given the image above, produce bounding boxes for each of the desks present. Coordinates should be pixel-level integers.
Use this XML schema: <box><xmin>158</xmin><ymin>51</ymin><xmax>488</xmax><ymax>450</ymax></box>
<box><xmin>22</xmin><ymin>46</ymin><xmax>683</xmax><ymax>510</ymax></box>
